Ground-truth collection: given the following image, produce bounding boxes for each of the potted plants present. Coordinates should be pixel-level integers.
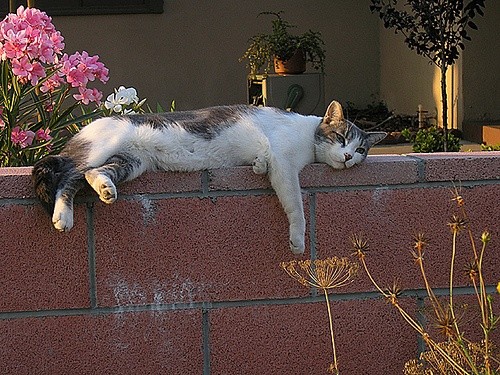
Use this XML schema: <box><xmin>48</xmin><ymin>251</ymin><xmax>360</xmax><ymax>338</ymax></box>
<box><xmin>237</xmin><ymin>9</ymin><xmax>329</xmax><ymax>94</ymax></box>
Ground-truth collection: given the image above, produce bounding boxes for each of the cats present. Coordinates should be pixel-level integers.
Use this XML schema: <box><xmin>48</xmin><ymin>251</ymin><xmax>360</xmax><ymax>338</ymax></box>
<box><xmin>32</xmin><ymin>100</ymin><xmax>389</xmax><ymax>257</ymax></box>
